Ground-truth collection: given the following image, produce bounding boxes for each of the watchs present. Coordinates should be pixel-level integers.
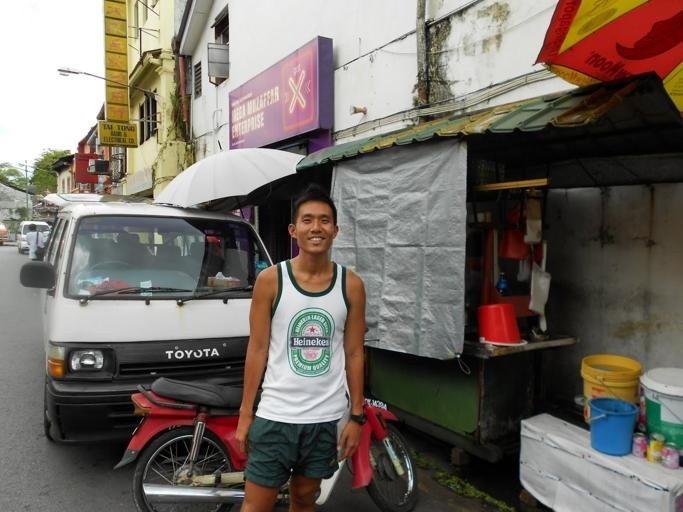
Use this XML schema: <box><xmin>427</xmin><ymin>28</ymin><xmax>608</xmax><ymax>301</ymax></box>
<box><xmin>348</xmin><ymin>413</ymin><xmax>367</xmax><ymax>426</ymax></box>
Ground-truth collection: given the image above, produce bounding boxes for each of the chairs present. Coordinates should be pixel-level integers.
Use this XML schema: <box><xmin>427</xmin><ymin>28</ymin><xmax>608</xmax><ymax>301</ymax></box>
<box><xmin>88</xmin><ymin>240</ymin><xmax>218</xmax><ymax>271</ymax></box>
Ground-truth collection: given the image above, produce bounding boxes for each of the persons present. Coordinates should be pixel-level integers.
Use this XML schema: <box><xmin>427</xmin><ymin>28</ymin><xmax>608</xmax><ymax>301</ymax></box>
<box><xmin>25</xmin><ymin>223</ymin><xmax>45</xmax><ymax>261</ymax></box>
<box><xmin>236</xmin><ymin>183</ymin><xmax>370</xmax><ymax>511</ymax></box>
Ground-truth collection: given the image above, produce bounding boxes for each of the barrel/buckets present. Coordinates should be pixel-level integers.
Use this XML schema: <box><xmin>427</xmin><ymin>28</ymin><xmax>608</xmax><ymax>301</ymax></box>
<box><xmin>588</xmin><ymin>397</ymin><xmax>638</xmax><ymax>455</ymax></box>
<box><xmin>477</xmin><ymin>303</ymin><xmax>528</xmax><ymax>348</ymax></box>
<box><xmin>638</xmin><ymin>367</ymin><xmax>683</xmax><ymax>456</ymax></box>
<box><xmin>579</xmin><ymin>354</ymin><xmax>642</xmax><ymax>424</ymax></box>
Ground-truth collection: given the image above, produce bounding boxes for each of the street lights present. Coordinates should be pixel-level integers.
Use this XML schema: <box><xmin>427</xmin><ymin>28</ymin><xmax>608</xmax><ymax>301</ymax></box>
<box><xmin>18</xmin><ymin>163</ymin><xmax>28</xmax><ymax>208</ymax></box>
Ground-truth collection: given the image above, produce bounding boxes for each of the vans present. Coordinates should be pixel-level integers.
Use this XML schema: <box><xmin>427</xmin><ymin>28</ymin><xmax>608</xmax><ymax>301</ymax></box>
<box><xmin>20</xmin><ymin>193</ymin><xmax>274</xmax><ymax>446</ymax></box>
<box><xmin>17</xmin><ymin>220</ymin><xmax>53</xmax><ymax>254</ymax></box>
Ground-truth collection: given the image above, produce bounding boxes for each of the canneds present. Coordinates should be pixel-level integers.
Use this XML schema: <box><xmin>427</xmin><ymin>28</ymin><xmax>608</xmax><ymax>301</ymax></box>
<box><xmin>633</xmin><ymin>430</ymin><xmax>679</xmax><ymax>469</ymax></box>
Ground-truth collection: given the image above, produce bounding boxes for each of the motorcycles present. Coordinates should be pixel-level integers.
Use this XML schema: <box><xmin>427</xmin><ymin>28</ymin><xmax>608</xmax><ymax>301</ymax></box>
<box><xmin>114</xmin><ymin>329</ymin><xmax>418</xmax><ymax>512</ymax></box>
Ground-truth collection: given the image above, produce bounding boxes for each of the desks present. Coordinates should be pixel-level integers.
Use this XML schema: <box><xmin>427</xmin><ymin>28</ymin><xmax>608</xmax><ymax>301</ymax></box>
<box><xmin>517</xmin><ymin>406</ymin><xmax>682</xmax><ymax>512</ymax></box>
